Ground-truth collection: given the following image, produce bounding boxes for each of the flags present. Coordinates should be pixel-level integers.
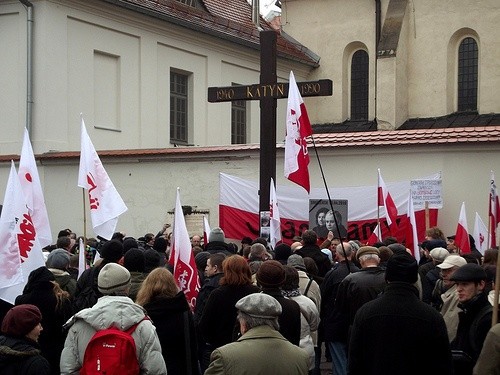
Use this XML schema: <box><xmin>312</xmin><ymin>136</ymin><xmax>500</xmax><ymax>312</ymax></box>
<box><xmin>270</xmin><ymin>180</ymin><xmax>281</xmax><ymax>250</ymax></box>
<box><xmin>203</xmin><ymin>217</ymin><xmax>210</xmax><ymax>246</ymax></box>
<box><xmin>167</xmin><ymin>190</ymin><xmax>201</xmax><ymax>313</ymax></box>
<box><xmin>18</xmin><ymin>128</ymin><xmax>52</xmax><ymax>249</ymax></box>
<box><xmin>0</xmin><ymin>162</ymin><xmax>46</xmax><ymax>305</ymax></box>
<box><xmin>455</xmin><ymin>204</ymin><xmax>470</xmax><ymax>253</ymax></box>
<box><xmin>489</xmin><ymin>174</ymin><xmax>500</xmax><ymax>248</ymax></box>
<box><xmin>78</xmin><ymin>119</ymin><xmax>128</xmax><ymax>240</ymax></box>
<box><xmin>473</xmin><ymin>212</ymin><xmax>491</xmax><ymax>256</ymax></box>
<box><xmin>367</xmin><ymin>173</ymin><xmax>420</xmax><ymax>264</ymax></box>
<box><xmin>284</xmin><ymin>71</ymin><xmax>313</xmax><ymax>194</ymax></box>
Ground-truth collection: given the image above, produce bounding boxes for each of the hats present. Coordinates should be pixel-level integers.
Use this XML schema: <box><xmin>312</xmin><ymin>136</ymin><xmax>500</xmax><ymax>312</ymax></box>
<box><xmin>46</xmin><ymin>248</ymin><xmax>72</xmax><ymax>268</ymax></box>
<box><xmin>209</xmin><ymin>227</ymin><xmax>225</xmax><ymax>242</ymax></box>
<box><xmin>429</xmin><ymin>247</ymin><xmax>449</xmax><ymax>262</ymax></box>
<box><xmin>437</xmin><ymin>254</ymin><xmax>467</xmax><ymax>269</ymax></box>
<box><xmin>355</xmin><ymin>246</ymin><xmax>380</xmax><ymax>262</ymax></box>
<box><xmin>99</xmin><ymin>237</ymin><xmax>168</xmax><ymax>272</ymax></box>
<box><xmin>447</xmin><ymin>235</ymin><xmax>456</xmax><ymax>240</ymax></box>
<box><xmin>448</xmin><ymin>263</ymin><xmax>488</xmax><ymax>281</ymax></box>
<box><xmin>287</xmin><ymin>254</ymin><xmax>307</xmax><ymax>270</ymax></box>
<box><xmin>385</xmin><ymin>250</ymin><xmax>418</xmax><ymax>283</ymax></box>
<box><xmin>235</xmin><ymin>291</ymin><xmax>282</xmax><ymax>318</ymax></box>
<box><xmin>256</xmin><ymin>261</ymin><xmax>287</xmax><ymax>286</ymax></box>
<box><xmin>2</xmin><ymin>304</ymin><xmax>41</xmax><ymax>337</ymax></box>
<box><xmin>27</xmin><ymin>266</ymin><xmax>55</xmax><ymax>284</ymax></box>
<box><xmin>98</xmin><ymin>263</ymin><xmax>131</xmax><ymax>293</ymax></box>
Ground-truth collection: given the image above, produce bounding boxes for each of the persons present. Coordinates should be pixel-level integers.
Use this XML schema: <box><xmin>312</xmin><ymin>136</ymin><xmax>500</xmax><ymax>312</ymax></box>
<box><xmin>0</xmin><ymin>227</ymin><xmax>500</xmax><ymax>375</ymax></box>
<box><xmin>312</xmin><ymin>208</ymin><xmax>329</xmax><ymax>237</ymax></box>
<box><xmin>325</xmin><ymin>210</ymin><xmax>347</xmax><ymax>236</ymax></box>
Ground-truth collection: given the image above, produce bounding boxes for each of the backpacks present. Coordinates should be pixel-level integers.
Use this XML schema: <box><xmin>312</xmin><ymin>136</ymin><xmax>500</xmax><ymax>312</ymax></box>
<box><xmin>79</xmin><ymin>316</ymin><xmax>150</xmax><ymax>375</ymax></box>
<box><xmin>72</xmin><ymin>266</ymin><xmax>98</xmax><ymax>315</ymax></box>
